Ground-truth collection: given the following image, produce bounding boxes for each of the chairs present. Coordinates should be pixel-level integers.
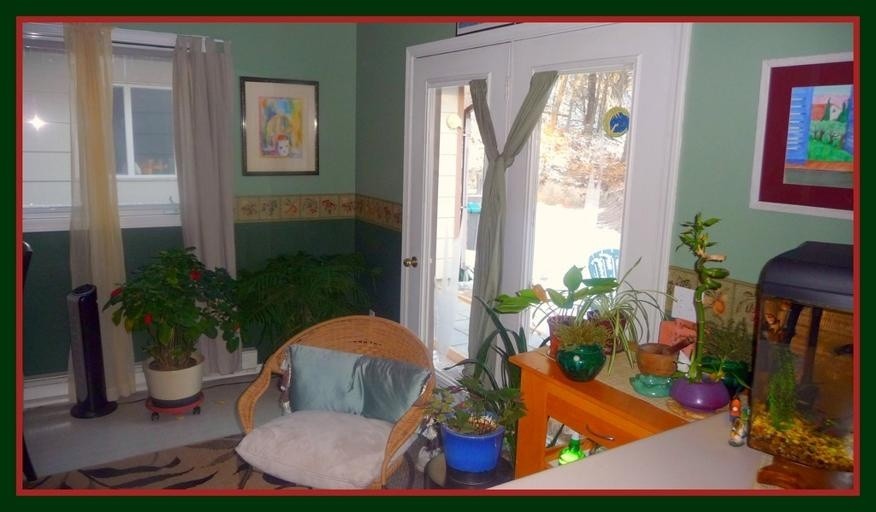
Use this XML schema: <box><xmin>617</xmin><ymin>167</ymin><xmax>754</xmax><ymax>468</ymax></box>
<box><xmin>233</xmin><ymin>314</ymin><xmax>434</xmax><ymax>489</ymax></box>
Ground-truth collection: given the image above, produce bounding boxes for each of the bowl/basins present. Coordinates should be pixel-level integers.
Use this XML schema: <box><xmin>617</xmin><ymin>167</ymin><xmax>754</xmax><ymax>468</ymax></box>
<box><xmin>636</xmin><ymin>342</ymin><xmax>679</xmax><ymax>377</ymax></box>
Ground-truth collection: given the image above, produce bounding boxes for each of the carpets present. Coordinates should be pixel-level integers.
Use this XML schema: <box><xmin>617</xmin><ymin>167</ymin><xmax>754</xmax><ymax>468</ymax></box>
<box><xmin>23</xmin><ymin>434</ymin><xmax>292</xmax><ymax>491</ymax></box>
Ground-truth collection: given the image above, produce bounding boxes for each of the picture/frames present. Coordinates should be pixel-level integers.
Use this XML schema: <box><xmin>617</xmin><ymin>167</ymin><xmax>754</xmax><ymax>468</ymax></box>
<box><xmin>748</xmin><ymin>54</ymin><xmax>859</xmax><ymax>217</ymax></box>
<box><xmin>240</xmin><ymin>76</ymin><xmax>320</xmax><ymax>174</ymax></box>
<box><xmin>455</xmin><ymin>22</ymin><xmax>518</xmax><ymax>36</ymax></box>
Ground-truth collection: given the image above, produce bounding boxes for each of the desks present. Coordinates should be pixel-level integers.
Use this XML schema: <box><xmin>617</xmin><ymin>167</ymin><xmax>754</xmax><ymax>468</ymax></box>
<box><xmin>509</xmin><ymin>331</ymin><xmax>735</xmax><ymax>479</ymax></box>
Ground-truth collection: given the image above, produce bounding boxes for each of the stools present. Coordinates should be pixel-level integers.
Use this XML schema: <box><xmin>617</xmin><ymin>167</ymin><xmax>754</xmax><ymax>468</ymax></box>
<box><xmin>425</xmin><ymin>452</ymin><xmax>513</xmax><ymax>491</ymax></box>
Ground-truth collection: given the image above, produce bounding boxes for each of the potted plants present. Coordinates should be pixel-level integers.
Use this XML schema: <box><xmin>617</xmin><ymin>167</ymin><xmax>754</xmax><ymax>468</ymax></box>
<box><xmin>702</xmin><ymin>315</ymin><xmax>752</xmax><ymax>394</ymax></box>
<box><xmin>492</xmin><ymin>263</ymin><xmax>615</xmax><ymax>357</ymax></box>
<box><xmin>670</xmin><ymin>211</ymin><xmax>735</xmax><ymax>412</ymax></box>
<box><xmin>582</xmin><ymin>271</ymin><xmax>679</xmax><ymax>369</ymax></box>
<box><xmin>550</xmin><ymin>321</ymin><xmax>607</xmax><ymax>382</ymax></box>
<box><xmin>427</xmin><ymin>377</ymin><xmax>527</xmax><ymax>473</ymax></box>
<box><xmin>103</xmin><ymin>246</ymin><xmax>244</xmax><ymax>419</ymax></box>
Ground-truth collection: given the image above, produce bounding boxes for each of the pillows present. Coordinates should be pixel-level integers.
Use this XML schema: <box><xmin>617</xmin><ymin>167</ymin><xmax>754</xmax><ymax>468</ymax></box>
<box><xmin>287</xmin><ymin>344</ymin><xmax>431</xmax><ymax>426</ymax></box>
